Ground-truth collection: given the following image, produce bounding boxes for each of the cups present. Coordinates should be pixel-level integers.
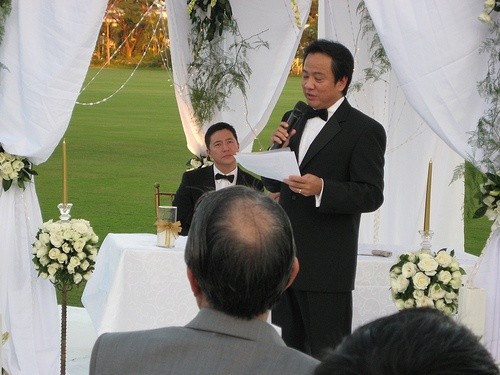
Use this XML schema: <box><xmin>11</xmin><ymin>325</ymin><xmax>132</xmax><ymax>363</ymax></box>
<box><xmin>157</xmin><ymin>205</ymin><xmax>177</xmax><ymax>247</ymax></box>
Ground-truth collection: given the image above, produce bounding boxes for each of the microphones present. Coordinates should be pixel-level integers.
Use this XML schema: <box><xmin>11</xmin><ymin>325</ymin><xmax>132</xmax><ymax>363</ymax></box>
<box><xmin>269</xmin><ymin>101</ymin><xmax>307</xmax><ymax>151</ymax></box>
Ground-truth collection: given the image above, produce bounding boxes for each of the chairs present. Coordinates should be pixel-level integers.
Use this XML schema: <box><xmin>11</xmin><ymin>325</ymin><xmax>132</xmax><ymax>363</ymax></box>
<box><xmin>154</xmin><ymin>182</ymin><xmax>176</xmax><ymax>232</ymax></box>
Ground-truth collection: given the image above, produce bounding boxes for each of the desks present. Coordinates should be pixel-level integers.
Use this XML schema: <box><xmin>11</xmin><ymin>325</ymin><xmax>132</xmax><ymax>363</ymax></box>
<box><xmin>81</xmin><ymin>233</ymin><xmax>199</xmax><ymax>328</ymax></box>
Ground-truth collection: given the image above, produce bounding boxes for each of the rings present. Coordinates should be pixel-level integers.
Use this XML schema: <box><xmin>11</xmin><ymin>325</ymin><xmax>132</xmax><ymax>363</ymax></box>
<box><xmin>298</xmin><ymin>189</ymin><xmax>302</xmax><ymax>194</ymax></box>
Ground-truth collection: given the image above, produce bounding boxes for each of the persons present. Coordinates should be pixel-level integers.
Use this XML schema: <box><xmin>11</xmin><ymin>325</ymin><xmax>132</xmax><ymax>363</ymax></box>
<box><xmin>88</xmin><ymin>185</ymin><xmax>322</xmax><ymax>375</ymax></box>
<box><xmin>171</xmin><ymin>122</ymin><xmax>265</xmax><ymax>237</ymax></box>
<box><xmin>260</xmin><ymin>39</ymin><xmax>388</xmax><ymax>361</ymax></box>
<box><xmin>314</xmin><ymin>308</ymin><xmax>500</xmax><ymax>375</ymax></box>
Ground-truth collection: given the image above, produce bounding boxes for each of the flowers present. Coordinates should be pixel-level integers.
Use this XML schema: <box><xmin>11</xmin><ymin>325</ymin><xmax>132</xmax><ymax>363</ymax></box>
<box><xmin>0</xmin><ymin>144</ymin><xmax>39</xmax><ymax>193</ymax></box>
<box><xmin>30</xmin><ymin>216</ymin><xmax>100</xmax><ymax>288</ymax></box>
<box><xmin>477</xmin><ymin>0</ymin><xmax>500</xmax><ymax>24</ymax></box>
<box><xmin>387</xmin><ymin>247</ymin><xmax>466</xmax><ymax>317</ymax></box>
<box><xmin>470</xmin><ymin>173</ymin><xmax>500</xmax><ymax>223</ymax></box>
<box><xmin>184</xmin><ymin>154</ymin><xmax>214</xmax><ymax>172</ymax></box>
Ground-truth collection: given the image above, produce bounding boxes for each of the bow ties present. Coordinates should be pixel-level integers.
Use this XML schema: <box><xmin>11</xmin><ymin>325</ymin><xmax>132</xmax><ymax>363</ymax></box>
<box><xmin>215</xmin><ymin>173</ymin><xmax>234</xmax><ymax>183</ymax></box>
<box><xmin>306</xmin><ymin>105</ymin><xmax>328</xmax><ymax>121</ymax></box>
